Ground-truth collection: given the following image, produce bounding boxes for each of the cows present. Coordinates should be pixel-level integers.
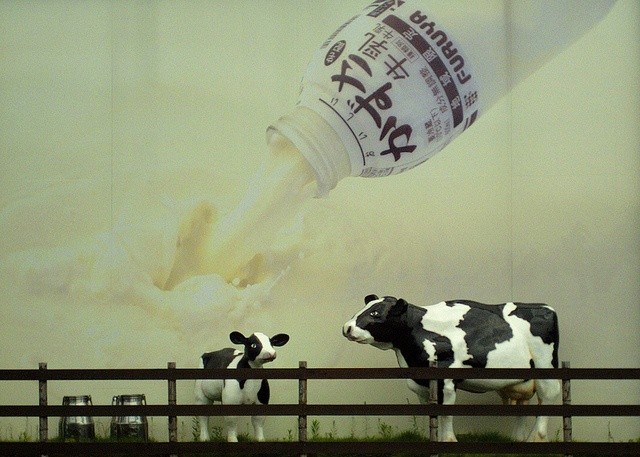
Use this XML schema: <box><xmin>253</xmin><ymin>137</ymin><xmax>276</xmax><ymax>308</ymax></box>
<box><xmin>342</xmin><ymin>295</ymin><xmax>561</xmax><ymax>441</ymax></box>
<box><xmin>195</xmin><ymin>331</ymin><xmax>290</xmax><ymax>443</ymax></box>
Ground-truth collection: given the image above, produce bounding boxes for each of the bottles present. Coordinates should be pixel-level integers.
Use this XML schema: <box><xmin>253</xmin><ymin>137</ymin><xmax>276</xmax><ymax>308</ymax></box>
<box><xmin>267</xmin><ymin>2</ymin><xmax>619</xmax><ymax>199</ymax></box>
